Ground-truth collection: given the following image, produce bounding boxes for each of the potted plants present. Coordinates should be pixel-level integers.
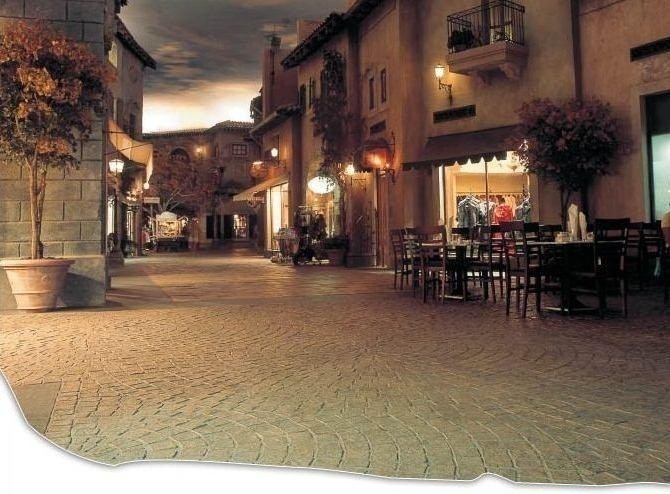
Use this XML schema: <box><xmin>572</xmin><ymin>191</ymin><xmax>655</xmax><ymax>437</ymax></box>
<box><xmin>446</xmin><ymin>26</ymin><xmax>476</xmax><ymax>50</ymax></box>
<box><xmin>322</xmin><ymin>235</ymin><xmax>349</xmax><ymax>266</ymax></box>
<box><xmin>0</xmin><ymin>14</ymin><xmax>119</xmax><ymax>313</ymax></box>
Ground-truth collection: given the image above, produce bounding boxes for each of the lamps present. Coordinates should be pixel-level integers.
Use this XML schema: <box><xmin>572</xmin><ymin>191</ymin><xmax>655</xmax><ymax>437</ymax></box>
<box><xmin>434</xmin><ymin>63</ymin><xmax>453</xmax><ymax>104</ymax></box>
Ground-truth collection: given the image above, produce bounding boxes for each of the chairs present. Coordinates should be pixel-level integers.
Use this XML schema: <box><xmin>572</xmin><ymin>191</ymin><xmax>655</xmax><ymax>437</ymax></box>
<box><xmin>388</xmin><ymin>217</ymin><xmax>670</xmax><ymax>325</ymax></box>
